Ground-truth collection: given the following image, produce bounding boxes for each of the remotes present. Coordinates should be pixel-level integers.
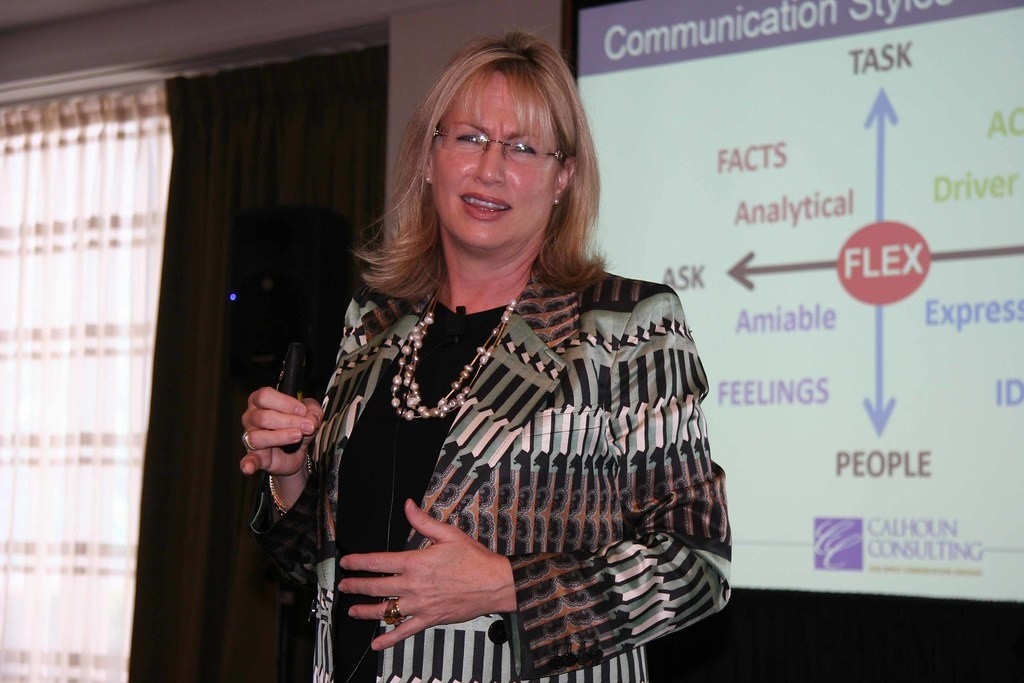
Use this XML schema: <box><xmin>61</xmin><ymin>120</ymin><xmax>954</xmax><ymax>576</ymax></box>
<box><xmin>276</xmin><ymin>343</ymin><xmax>308</xmax><ymax>455</ymax></box>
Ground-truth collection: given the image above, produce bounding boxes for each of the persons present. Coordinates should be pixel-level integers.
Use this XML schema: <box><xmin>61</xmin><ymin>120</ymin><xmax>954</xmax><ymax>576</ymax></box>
<box><xmin>236</xmin><ymin>25</ymin><xmax>733</xmax><ymax>682</ymax></box>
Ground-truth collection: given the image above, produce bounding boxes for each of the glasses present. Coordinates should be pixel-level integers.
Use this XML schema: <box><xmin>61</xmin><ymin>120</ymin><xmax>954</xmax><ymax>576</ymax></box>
<box><xmin>436</xmin><ymin>123</ymin><xmax>573</xmax><ymax>167</ymax></box>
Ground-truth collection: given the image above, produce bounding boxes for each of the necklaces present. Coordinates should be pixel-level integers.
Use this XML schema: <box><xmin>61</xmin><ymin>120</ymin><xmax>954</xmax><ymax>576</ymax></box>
<box><xmin>392</xmin><ymin>276</ymin><xmax>529</xmax><ymax>420</ymax></box>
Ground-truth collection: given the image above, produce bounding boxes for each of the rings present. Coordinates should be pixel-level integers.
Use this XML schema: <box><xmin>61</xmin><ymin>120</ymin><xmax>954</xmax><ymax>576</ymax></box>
<box><xmin>384</xmin><ymin>598</ymin><xmax>405</xmax><ymax>625</ymax></box>
<box><xmin>241</xmin><ymin>428</ymin><xmax>255</xmax><ymax>452</ymax></box>
